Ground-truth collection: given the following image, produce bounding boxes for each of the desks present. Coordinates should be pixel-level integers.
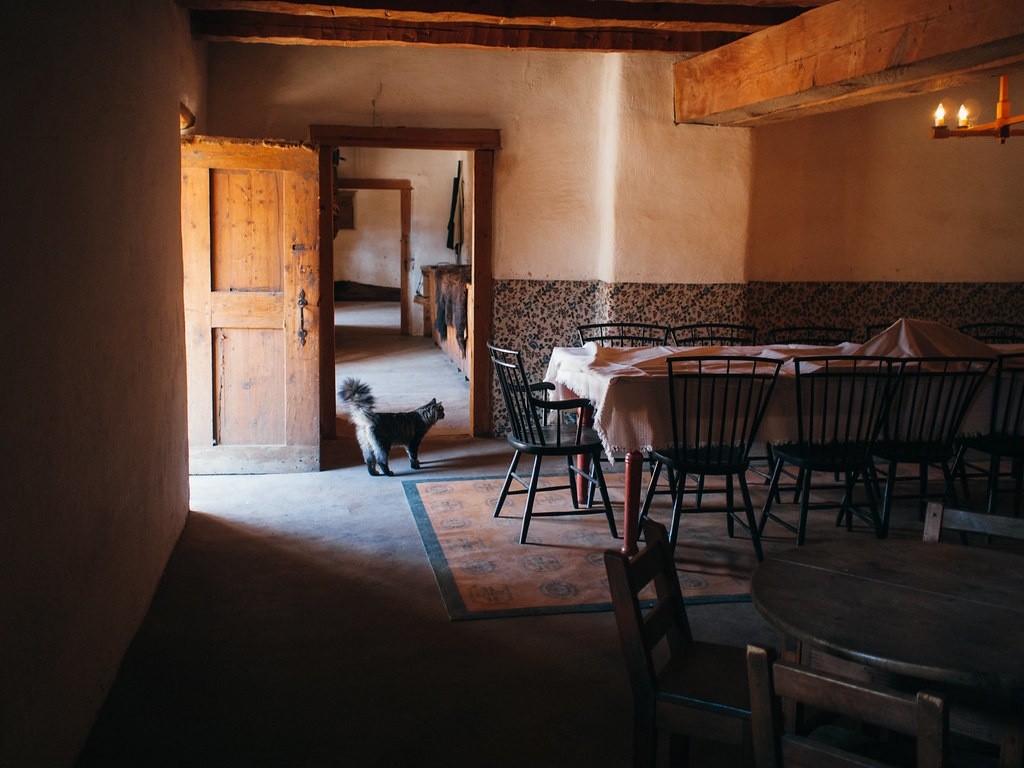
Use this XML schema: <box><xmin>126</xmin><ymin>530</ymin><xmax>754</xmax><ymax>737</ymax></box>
<box><xmin>747</xmin><ymin>538</ymin><xmax>1024</xmax><ymax>768</ymax></box>
<box><xmin>542</xmin><ymin>342</ymin><xmax>1024</xmax><ymax>560</ymax></box>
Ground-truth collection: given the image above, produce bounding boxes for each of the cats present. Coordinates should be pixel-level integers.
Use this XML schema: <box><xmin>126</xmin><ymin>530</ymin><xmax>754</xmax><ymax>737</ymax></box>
<box><xmin>339</xmin><ymin>378</ymin><xmax>445</xmax><ymax>476</ymax></box>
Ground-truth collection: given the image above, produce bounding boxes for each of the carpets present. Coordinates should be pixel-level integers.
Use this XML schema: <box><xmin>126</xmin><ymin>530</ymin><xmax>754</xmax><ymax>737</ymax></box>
<box><xmin>400</xmin><ymin>460</ymin><xmax>1024</xmax><ymax>623</ymax></box>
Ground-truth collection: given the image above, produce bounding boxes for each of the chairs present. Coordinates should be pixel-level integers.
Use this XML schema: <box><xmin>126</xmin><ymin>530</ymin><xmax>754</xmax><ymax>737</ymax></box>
<box><xmin>485</xmin><ymin>321</ymin><xmax>1024</xmax><ymax>565</ymax></box>
<box><xmin>748</xmin><ymin>642</ymin><xmax>999</xmax><ymax>768</ymax></box>
<box><xmin>922</xmin><ymin>498</ymin><xmax>1024</xmax><ymax>551</ymax></box>
<box><xmin>603</xmin><ymin>514</ymin><xmax>749</xmax><ymax>768</ymax></box>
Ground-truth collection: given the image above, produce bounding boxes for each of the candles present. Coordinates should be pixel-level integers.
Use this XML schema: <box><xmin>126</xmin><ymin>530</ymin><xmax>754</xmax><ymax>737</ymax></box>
<box><xmin>932</xmin><ymin>103</ymin><xmax>946</xmax><ymax>126</ymax></box>
<box><xmin>958</xmin><ymin>103</ymin><xmax>969</xmax><ymax>127</ymax></box>
